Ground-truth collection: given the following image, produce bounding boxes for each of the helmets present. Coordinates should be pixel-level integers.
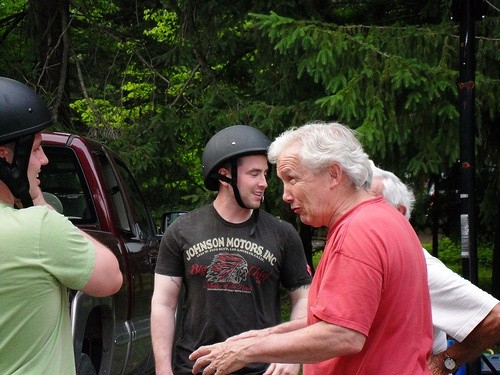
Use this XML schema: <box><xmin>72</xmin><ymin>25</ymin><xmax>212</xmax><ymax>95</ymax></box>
<box><xmin>202</xmin><ymin>125</ymin><xmax>272</xmax><ymax>191</ymax></box>
<box><xmin>0</xmin><ymin>77</ymin><xmax>55</xmax><ymax>145</ymax></box>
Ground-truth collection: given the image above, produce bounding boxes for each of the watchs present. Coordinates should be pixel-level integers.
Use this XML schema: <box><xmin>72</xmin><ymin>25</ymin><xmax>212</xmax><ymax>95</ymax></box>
<box><xmin>439</xmin><ymin>353</ymin><xmax>459</xmax><ymax>373</ymax></box>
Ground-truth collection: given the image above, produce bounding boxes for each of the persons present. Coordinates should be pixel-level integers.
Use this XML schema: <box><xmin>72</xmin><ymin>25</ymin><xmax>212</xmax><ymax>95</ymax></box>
<box><xmin>372</xmin><ymin>167</ymin><xmax>500</xmax><ymax>375</ymax></box>
<box><xmin>0</xmin><ymin>77</ymin><xmax>123</xmax><ymax>375</ymax></box>
<box><xmin>150</xmin><ymin>125</ymin><xmax>313</xmax><ymax>375</ymax></box>
<box><xmin>189</xmin><ymin>123</ymin><xmax>434</xmax><ymax>375</ymax></box>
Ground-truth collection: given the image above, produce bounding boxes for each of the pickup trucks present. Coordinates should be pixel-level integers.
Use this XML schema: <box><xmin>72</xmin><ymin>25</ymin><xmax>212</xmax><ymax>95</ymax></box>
<box><xmin>39</xmin><ymin>129</ymin><xmax>190</xmax><ymax>375</ymax></box>
